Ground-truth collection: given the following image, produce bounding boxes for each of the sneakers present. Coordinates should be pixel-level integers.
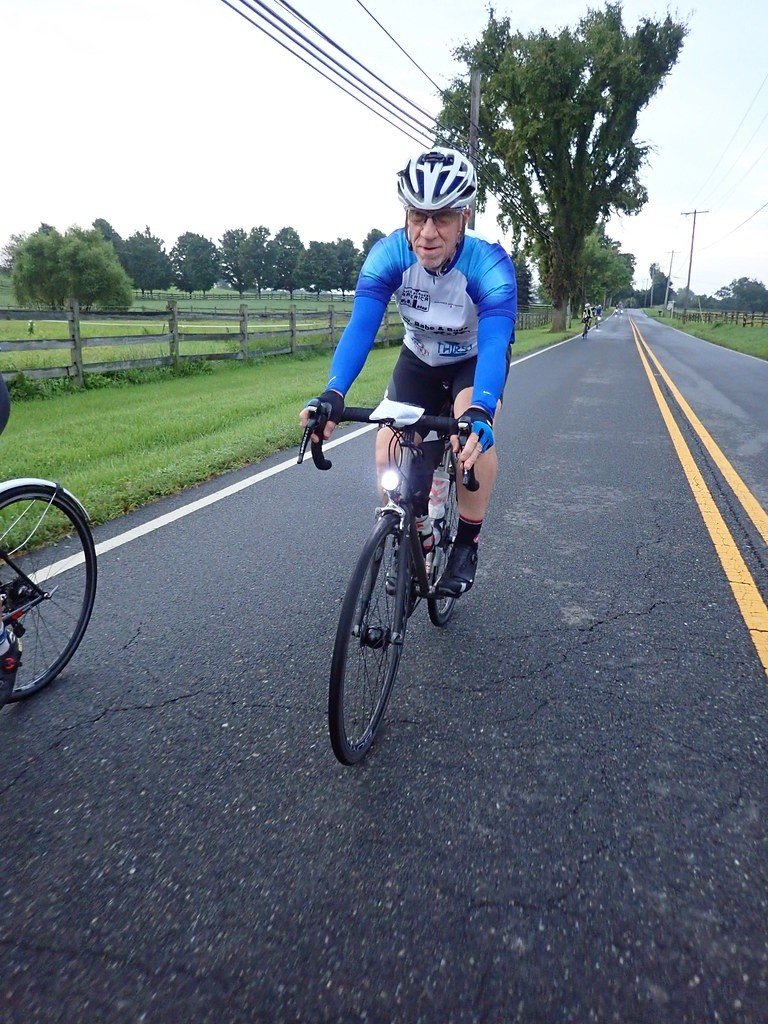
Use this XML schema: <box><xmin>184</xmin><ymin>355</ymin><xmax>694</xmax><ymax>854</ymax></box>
<box><xmin>0</xmin><ymin>639</ymin><xmax>22</xmax><ymax>709</ymax></box>
<box><xmin>439</xmin><ymin>542</ymin><xmax>478</xmax><ymax>595</ymax></box>
<box><xmin>386</xmin><ymin>556</ymin><xmax>398</xmax><ymax>595</ymax></box>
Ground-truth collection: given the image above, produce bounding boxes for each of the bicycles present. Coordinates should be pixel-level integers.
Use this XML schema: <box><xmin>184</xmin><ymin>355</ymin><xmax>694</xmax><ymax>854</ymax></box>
<box><xmin>594</xmin><ymin>314</ymin><xmax>602</xmax><ymax>329</ymax></box>
<box><xmin>296</xmin><ymin>399</ymin><xmax>482</xmax><ymax>768</ymax></box>
<box><xmin>581</xmin><ymin>317</ymin><xmax>593</xmax><ymax>340</ymax></box>
<box><xmin>0</xmin><ymin>475</ymin><xmax>99</xmax><ymax>706</ymax></box>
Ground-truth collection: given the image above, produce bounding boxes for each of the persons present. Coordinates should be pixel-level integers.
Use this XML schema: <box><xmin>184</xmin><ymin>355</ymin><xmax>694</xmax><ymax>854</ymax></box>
<box><xmin>0</xmin><ymin>371</ymin><xmax>23</xmax><ymax>709</ymax></box>
<box><xmin>298</xmin><ymin>146</ymin><xmax>517</xmax><ymax>600</ymax></box>
<box><xmin>581</xmin><ymin>302</ymin><xmax>602</xmax><ymax>338</ymax></box>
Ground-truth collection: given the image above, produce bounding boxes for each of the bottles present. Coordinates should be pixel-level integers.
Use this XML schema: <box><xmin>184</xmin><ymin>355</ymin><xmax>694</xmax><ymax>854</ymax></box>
<box><xmin>415</xmin><ymin>514</ymin><xmax>434</xmax><ymax>549</ymax></box>
<box><xmin>428</xmin><ymin>464</ymin><xmax>450</xmax><ymax>518</ymax></box>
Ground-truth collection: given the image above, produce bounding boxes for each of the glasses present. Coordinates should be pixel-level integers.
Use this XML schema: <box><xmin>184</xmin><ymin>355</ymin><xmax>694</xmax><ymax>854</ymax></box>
<box><xmin>403</xmin><ymin>205</ymin><xmax>467</xmax><ymax>227</ymax></box>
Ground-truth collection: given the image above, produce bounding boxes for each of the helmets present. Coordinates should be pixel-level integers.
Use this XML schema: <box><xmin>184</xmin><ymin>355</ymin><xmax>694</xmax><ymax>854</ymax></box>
<box><xmin>398</xmin><ymin>147</ymin><xmax>477</xmax><ymax>210</ymax></box>
